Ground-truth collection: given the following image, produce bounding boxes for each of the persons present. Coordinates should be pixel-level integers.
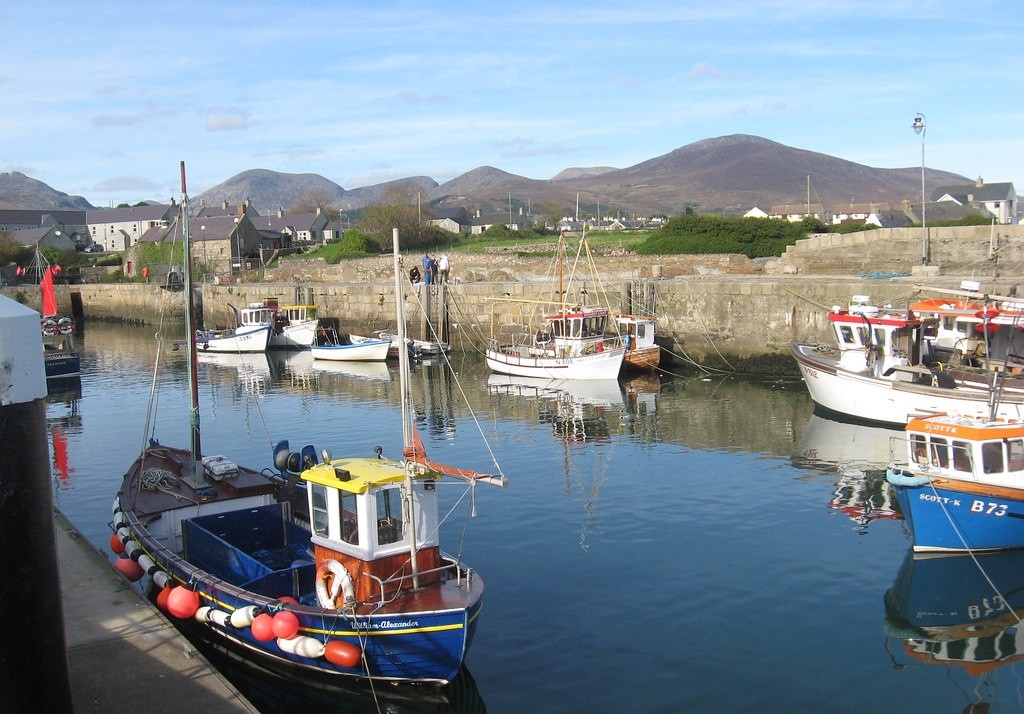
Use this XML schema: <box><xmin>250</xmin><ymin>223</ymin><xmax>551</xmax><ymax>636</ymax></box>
<box><xmin>276</xmin><ymin>311</ymin><xmax>285</xmax><ymax>330</ymax></box>
<box><xmin>410</xmin><ymin>266</ymin><xmax>421</xmax><ymax>287</ymax></box>
<box><xmin>422</xmin><ymin>252</ymin><xmax>433</xmax><ymax>285</ymax></box>
<box><xmin>437</xmin><ymin>253</ymin><xmax>450</xmax><ymax>286</ymax></box>
<box><xmin>428</xmin><ymin>254</ymin><xmax>439</xmax><ymax>285</ymax></box>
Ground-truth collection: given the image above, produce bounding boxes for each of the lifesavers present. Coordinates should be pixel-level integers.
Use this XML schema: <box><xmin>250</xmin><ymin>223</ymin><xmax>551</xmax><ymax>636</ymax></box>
<box><xmin>316</xmin><ymin>558</ymin><xmax>356</xmax><ymax>610</ymax></box>
<box><xmin>42</xmin><ymin>320</ymin><xmax>58</xmax><ymax>338</ymax></box>
<box><xmin>58</xmin><ymin>317</ymin><xmax>74</xmax><ymax>334</ymax></box>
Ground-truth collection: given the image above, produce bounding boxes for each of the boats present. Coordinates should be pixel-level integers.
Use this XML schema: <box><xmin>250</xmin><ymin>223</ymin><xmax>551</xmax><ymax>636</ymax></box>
<box><xmin>885</xmin><ymin>548</ymin><xmax>1024</xmax><ymax>674</ymax></box>
<box><xmin>18</xmin><ymin>242</ymin><xmax>80</xmax><ymax>378</ymax></box>
<box><xmin>886</xmin><ymin>308</ymin><xmax>1023</xmax><ymax>553</ymax></box>
<box><xmin>564</xmin><ymin>226</ymin><xmax>660</xmax><ymax>371</ymax></box>
<box><xmin>379</xmin><ymin>332</ymin><xmax>447</xmax><ymax>354</ymax></box>
<box><xmin>310</xmin><ymin>325</ymin><xmax>392</xmax><ymax>362</ymax></box>
<box><xmin>212</xmin><ymin>659</ymin><xmax>485</xmax><ymax>714</ymax></box>
<box><xmin>112</xmin><ymin>161</ymin><xmax>507</xmax><ymax>696</ymax></box>
<box><xmin>486</xmin><ymin>232</ymin><xmax>626</xmax><ymax>382</ymax></box>
<box><xmin>195</xmin><ymin>303</ymin><xmax>273</xmax><ymax>353</ymax></box>
<box><xmin>349</xmin><ymin>334</ymin><xmax>423</xmax><ymax>359</ymax></box>
<box><xmin>791</xmin><ymin>235</ymin><xmax>1022</xmax><ymax>424</ymax></box>
<box><xmin>216</xmin><ymin>298</ymin><xmax>320</xmax><ymax>349</ymax></box>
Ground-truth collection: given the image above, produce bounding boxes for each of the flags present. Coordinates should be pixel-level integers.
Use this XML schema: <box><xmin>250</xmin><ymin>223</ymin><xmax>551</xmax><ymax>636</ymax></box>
<box><xmin>51</xmin><ymin>263</ymin><xmax>62</xmax><ymax>276</ymax></box>
<box><xmin>40</xmin><ymin>266</ymin><xmax>58</xmax><ymax>318</ymax></box>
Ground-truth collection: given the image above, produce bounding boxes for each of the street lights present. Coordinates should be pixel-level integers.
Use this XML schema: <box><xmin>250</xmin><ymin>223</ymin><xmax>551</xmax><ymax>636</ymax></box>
<box><xmin>911</xmin><ymin>112</ymin><xmax>927</xmax><ymax>265</ymax></box>
<box><xmin>201</xmin><ymin>225</ymin><xmax>207</xmax><ymax>273</ymax></box>
<box><xmin>234</xmin><ymin>217</ymin><xmax>240</xmax><ymax>271</ymax></box>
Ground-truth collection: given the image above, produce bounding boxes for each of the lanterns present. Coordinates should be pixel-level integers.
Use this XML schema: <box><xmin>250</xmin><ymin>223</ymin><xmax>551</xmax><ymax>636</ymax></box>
<box><xmin>1018</xmin><ymin>323</ymin><xmax>1024</xmax><ymax>330</ymax></box>
<box><xmin>975</xmin><ymin>322</ymin><xmax>1000</xmax><ymax>334</ymax></box>
<box><xmin>974</xmin><ymin>310</ymin><xmax>999</xmax><ymax>322</ymax></box>
